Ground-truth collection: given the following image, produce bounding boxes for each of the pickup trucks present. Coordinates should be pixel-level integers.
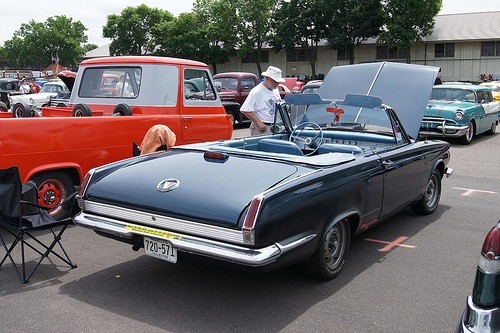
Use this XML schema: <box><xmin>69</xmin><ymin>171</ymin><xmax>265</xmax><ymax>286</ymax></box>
<box><xmin>0</xmin><ymin>56</ymin><xmax>234</xmax><ymax>231</ymax></box>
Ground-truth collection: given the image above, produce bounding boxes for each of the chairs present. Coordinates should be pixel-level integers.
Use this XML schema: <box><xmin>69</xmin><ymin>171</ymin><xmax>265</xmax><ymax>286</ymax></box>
<box><xmin>0</xmin><ymin>164</ymin><xmax>78</xmax><ymax>284</ymax></box>
<box><xmin>318</xmin><ymin>144</ymin><xmax>364</xmax><ymax>157</ymax></box>
<box><xmin>257</xmin><ymin>138</ymin><xmax>305</xmax><ymax>157</ymax></box>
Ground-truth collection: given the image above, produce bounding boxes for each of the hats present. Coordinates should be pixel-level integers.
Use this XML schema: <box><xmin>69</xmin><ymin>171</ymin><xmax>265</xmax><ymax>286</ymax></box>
<box><xmin>261</xmin><ymin>65</ymin><xmax>286</xmax><ymax>83</ymax></box>
<box><xmin>22</xmin><ymin>78</ymin><xmax>26</xmax><ymax>82</ymax></box>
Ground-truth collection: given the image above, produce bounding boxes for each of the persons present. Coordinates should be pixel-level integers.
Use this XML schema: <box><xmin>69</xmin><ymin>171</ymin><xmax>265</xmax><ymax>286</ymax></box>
<box><xmin>239</xmin><ymin>66</ymin><xmax>291</xmax><ymax>136</ymax></box>
<box><xmin>18</xmin><ymin>77</ymin><xmax>31</xmax><ymax>94</ymax></box>
<box><xmin>29</xmin><ymin>77</ymin><xmax>42</xmax><ymax>94</ymax></box>
<box><xmin>116</xmin><ymin>74</ymin><xmax>134</xmax><ymax>97</ymax></box>
<box><xmin>258</xmin><ymin>76</ymin><xmax>267</xmax><ymax>83</ymax></box>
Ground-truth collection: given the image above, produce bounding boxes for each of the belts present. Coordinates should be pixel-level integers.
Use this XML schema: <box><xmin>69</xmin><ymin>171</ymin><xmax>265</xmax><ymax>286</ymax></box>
<box><xmin>264</xmin><ymin>123</ymin><xmax>274</xmax><ymax>126</ymax></box>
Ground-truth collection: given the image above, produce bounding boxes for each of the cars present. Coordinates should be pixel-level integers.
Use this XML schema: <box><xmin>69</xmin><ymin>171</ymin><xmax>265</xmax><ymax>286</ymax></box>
<box><xmin>73</xmin><ymin>61</ymin><xmax>453</xmax><ymax>282</ymax></box>
<box><xmin>1</xmin><ymin>72</ymin><xmax>500</xmax><ymax>144</ymax></box>
